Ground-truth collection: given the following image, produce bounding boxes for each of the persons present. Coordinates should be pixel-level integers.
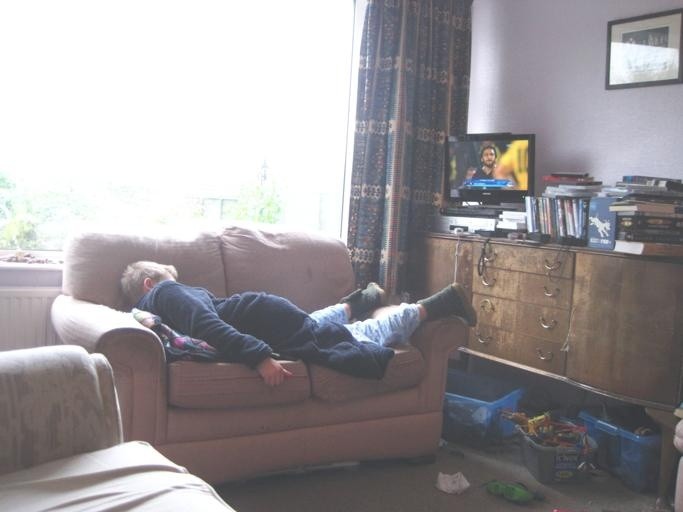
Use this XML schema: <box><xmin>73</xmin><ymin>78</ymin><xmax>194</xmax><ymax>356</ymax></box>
<box><xmin>472</xmin><ymin>146</ymin><xmax>500</xmax><ymax>190</ymax></box>
<box><xmin>494</xmin><ymin>140</ymin><xmax>528</xmax><ymax>190</ymax></box>
<box><xmin>120</xmin><ymin>261</ymin><xmax>476</xmax><ymax>385</ymax></box>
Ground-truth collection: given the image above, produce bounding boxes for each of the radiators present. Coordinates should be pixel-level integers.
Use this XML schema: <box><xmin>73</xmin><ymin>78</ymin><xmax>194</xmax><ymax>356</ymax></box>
<box><xmin>0</xmin><ymin>285</ymin><xmax>61</xmax><ymax>350</ymax></box>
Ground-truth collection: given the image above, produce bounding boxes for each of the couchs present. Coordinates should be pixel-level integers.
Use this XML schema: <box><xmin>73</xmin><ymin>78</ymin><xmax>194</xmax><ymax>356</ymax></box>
<box><xmin>47</xmin><ymin>223</ymin><xmax>470</xmax><ymax>486</ymax></box>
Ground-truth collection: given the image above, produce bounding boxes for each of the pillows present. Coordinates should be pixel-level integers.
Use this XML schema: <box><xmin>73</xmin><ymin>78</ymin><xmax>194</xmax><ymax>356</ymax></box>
<box><xmin>131</xmin><ymin>307</ymin><xmax>223</xmax><ymax>366</ymax></box>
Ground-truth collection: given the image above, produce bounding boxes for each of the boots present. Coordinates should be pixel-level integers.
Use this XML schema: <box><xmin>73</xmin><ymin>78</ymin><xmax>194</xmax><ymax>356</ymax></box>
<box><xmin>338</xmin><ymin>279</ymin><xmax>390</xmax><ymax>323</ymax></box>
<box><xmin>414</xmin><ymin>279</ymin><xmax>480</xmax><ymax>329</ymax></box>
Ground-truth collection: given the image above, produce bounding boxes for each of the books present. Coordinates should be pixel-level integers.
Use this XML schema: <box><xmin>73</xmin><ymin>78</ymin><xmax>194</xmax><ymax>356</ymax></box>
<box><xmin>525</xmin><ymin>175</ymin><xmax>683</xmax><ymax>257</ymax></box>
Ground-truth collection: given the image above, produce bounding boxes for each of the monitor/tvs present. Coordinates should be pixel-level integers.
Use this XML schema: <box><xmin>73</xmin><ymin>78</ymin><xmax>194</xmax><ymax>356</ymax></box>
<box><xmin>444</xmin><ymin>133</ymin><xmax>535</xmax><ymax>211</ymax></box>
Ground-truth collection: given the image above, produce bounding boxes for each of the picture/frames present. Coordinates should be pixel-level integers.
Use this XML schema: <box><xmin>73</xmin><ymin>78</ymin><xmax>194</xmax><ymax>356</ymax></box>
<box><xmin>603</xmin><ymin>7</ymin><xmax>682</xmax><ymax>92</ymax></box>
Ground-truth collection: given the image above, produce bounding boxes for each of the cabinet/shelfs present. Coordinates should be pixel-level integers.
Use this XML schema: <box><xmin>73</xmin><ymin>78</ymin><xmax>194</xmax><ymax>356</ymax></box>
<box><xmin>417</xmin><ymin>228</ymin><xmax>682</xmax><ymax>512</ymax></box>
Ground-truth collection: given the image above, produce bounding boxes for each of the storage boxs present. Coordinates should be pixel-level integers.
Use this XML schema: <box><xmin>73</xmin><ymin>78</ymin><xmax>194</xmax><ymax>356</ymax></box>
<box><xmin>443</xmin><ymin>371</ymin><xmax>525</xmax><ymax>448</ymax></box>
<box><xmin>576</xmin><ymin>406</ymin><xmax>661</xmax><ymax>495</ymax></box>
<box><xmin>512</xmin><ymin>413</ymin><xmax>598</xmax><ymax>488</ymax></box>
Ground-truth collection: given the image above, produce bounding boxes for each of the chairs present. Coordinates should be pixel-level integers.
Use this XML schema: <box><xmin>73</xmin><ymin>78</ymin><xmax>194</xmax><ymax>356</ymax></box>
<box><xmin>0</xmin><ymin>341</ymin><xmax>239</xmax><ymax>512</ymax></box>
